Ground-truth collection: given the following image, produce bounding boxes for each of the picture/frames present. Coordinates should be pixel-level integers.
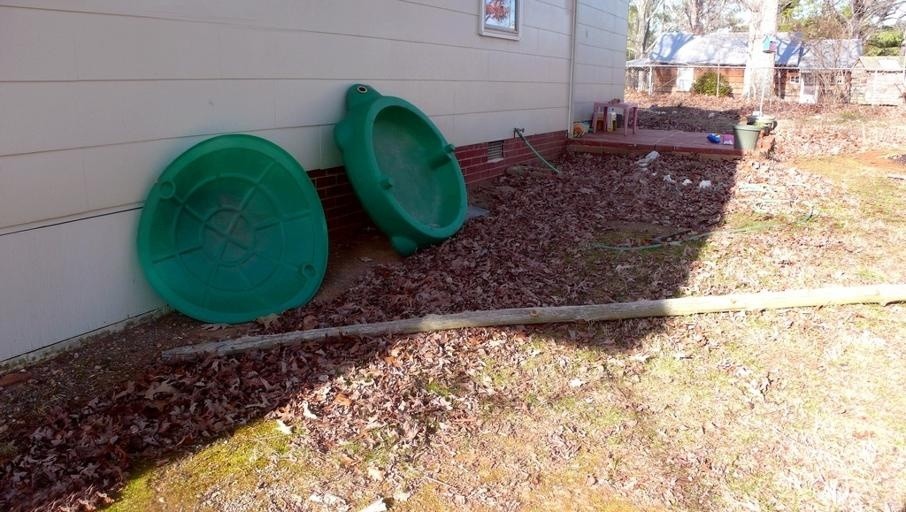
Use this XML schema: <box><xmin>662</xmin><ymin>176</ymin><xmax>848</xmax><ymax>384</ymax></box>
<box><xmin>479</xmin><ymin>0</ymin><xmax>523</xmax><ymax>41</ymax></box>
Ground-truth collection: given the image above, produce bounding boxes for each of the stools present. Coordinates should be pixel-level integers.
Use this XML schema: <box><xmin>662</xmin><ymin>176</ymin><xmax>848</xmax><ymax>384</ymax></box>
<box><xmin>592</xmin><ymin>101</ymin><xmax>638</xmax><ymax>136</ymax></box>
